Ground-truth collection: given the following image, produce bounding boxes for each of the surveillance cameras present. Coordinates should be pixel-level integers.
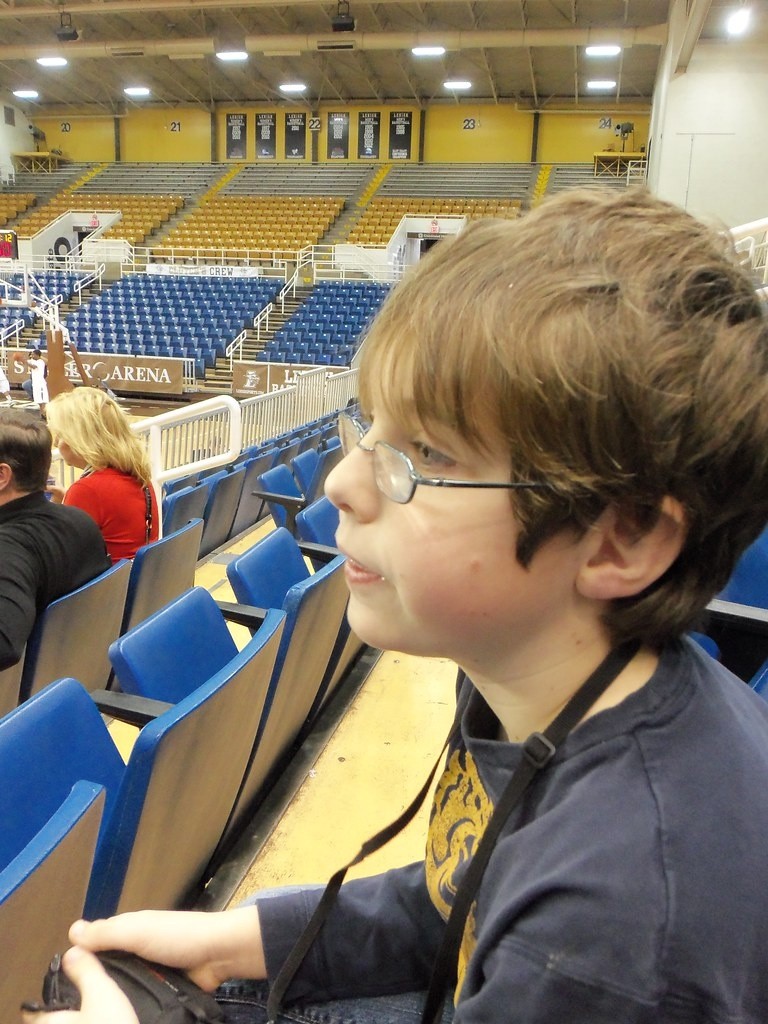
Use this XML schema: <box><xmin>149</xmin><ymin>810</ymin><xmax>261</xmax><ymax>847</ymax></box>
<box><xmin>615</xmin><ymin>123</ymin><xmax>634</xmax><ymax>133</ymax></box>
<box><xmin>28</xmin><ymin>124</ymin><xmax>38</xmax><ymax>133</ymax></box>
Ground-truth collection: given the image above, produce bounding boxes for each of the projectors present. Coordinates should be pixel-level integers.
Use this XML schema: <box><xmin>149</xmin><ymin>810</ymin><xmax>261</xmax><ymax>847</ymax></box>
<box><xmin>331</xmin><ymin>16</ymin><xmax>356</xmax><ymax>32</ymax></box>
<box><xmin>56</xmin><ymin>27</ymin><xmax>80</xmax><ymax>42</ymax></box>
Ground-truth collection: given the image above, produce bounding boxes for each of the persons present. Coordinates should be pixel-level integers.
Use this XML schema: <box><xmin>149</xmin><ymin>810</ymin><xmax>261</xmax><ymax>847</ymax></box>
<box><xmin>0</xmin><ymin>401</ymin><xmax>108</xmax><ymax>707</ymax></box>
<box><xmin>43</xmin><ymin>387</ymin><xmax>160</xmax><ymax>565</ymax></box>
<box><xmin>22</xmin><ymin>187</ymin><xmax>768</xmax><ymax>1024</ymax></box>
<box><xmin>13</xmin><ymin>348</ymin><xmax>50</xmax><ymax>419</ymax></box>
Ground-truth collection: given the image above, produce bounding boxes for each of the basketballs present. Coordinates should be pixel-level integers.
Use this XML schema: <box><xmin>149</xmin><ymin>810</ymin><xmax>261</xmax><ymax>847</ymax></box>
<box><xmin>12</xmin><ymin>352</ymin><xmax>23</xmax><ymax>362</ymax></box>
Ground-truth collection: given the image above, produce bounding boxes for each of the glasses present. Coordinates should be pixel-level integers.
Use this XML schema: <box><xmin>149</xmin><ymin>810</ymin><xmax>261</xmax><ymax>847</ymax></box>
<box><xmin>339</xmin><ymin>398</ymin><xmax>558</xmax><ymax>506</ymax></box>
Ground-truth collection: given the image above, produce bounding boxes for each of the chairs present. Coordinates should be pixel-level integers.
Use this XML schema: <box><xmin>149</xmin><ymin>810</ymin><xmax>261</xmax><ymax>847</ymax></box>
<box><xmin>0</xmin><ymin>196</ymin><xmax>766</xmax><ymax>1022</ymax></box>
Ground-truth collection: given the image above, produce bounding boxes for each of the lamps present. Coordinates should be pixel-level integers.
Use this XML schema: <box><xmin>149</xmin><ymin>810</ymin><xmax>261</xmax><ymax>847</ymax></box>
<box><xmin>57</xmin><ymin>10</ymin><xmax>79</xmax><ymax>43</ymax></box>
<box><xmin>332</xmin><ymin>1</ymin><xmax>357</xmax><ymax>32</ymax></box>
